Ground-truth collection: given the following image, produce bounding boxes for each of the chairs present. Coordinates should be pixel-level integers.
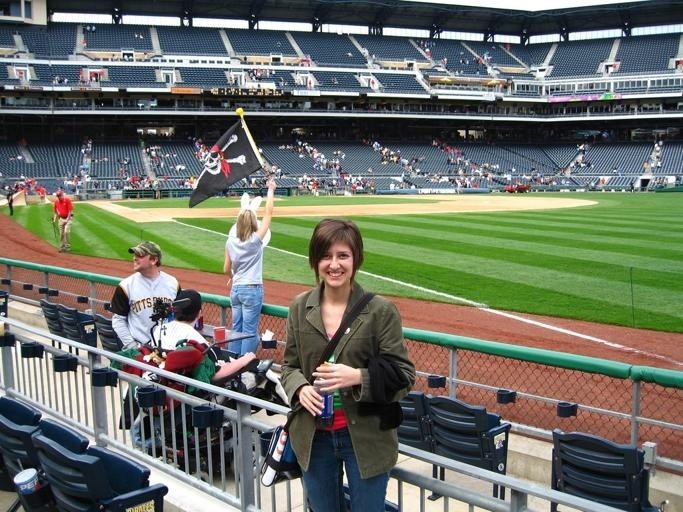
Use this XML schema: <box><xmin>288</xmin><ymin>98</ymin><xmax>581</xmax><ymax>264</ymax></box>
<box><xmin>396</xmin><ymin>386</ymin><xmax>669</xmax><ymax>512</ymax></box>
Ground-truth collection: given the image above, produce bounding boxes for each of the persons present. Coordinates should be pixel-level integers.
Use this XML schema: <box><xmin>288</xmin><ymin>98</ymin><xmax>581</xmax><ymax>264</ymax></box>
<box><xmin>6</xmin><ymin>192</ymin><xmax>17</xmax><ymax>217</ymax></box>
<box><xmin>151</xmin><ymin>289</ymin><xmax>257</xmax><ymax>390</ymax></box>
<box><xmin>109</xmin><ymin>239</ymin><xmax>186</xmax><ymax>428</ymax></box>
<box><xmin>528</xmin><ymin>139</ymin><xmax>681</xmax><ymax>196</ymax></box>
<box><xmin>221</xmin><ymin>176</ymin><xmax>277</xmax><ymax>357</ymax></box>
<box><xmin>1</xmin><ymin>137</ymin><xmax>200</xmax><ymax>197</ymax></box>
<box><xmin>276</xmin><ymin>216</ymin><xmax>416</xmax><ymax>512</ymax></box>
<box><xmin>33</xmin><ymin>184</ymin><xmax>47</xmax><ymax>203</ymax></box>
<box><xmin>242</xmin><ymin>128</ymin><xmax>526</xmax><ymax>196</ymax></box>
<box><xmin>414</xmin><ymin>38</ymin><xmax>499</xmax><ymax>77</ymax></box>
<box><xmin>50</xmin><ymin>188</ymin><xmax>73</xmax><ymax>254</ymax></box>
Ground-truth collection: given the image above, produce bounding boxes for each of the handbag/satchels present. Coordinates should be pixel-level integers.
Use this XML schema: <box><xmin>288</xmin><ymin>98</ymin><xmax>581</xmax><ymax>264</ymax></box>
<box><xmin>259</xmin><ymin>426</ymin><xmax>302</xmax><ymax>487</ymax></box>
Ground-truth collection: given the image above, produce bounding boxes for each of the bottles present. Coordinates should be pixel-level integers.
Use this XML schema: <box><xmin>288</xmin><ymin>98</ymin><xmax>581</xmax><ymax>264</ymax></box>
<box><xmin>312</xmin><ymin>362</ymin><xmax>335</xmax><ymax>428</ymax></box>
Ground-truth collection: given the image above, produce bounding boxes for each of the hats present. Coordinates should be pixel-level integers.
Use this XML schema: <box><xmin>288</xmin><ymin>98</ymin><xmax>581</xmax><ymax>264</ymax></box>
<box><xmin>56</xmin><ymin>191</ymin><xmax>62</xmax><ymax>196</ymax></box>
<box><xmin>173</xmin><ymin>289</ymin><xmax>201</xmax><ymax>309</ymax></box>
<box><xmin>129</xmin><ymin>240</ymin><xmax>161</xmax><ymax>257</ymax></box>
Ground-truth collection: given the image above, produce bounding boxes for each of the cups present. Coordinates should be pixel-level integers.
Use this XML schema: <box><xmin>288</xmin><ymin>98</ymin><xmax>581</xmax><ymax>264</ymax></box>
<box><xmin>13</xmin><ymin>468</ymin><xmax>42</xmax><ymax>496</ymax></box>
<box><xmin>213</xmin><ymin>327</ymin><xmax>226</xmax><ymax>348</ymax></box>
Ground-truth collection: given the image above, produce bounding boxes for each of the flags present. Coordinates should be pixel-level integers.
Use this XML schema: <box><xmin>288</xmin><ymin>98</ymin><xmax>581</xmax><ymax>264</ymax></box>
<box><xmin>189</xmin><ymin>120</ymin><xmax>263</xmax><ymax>209</ymax></box>
